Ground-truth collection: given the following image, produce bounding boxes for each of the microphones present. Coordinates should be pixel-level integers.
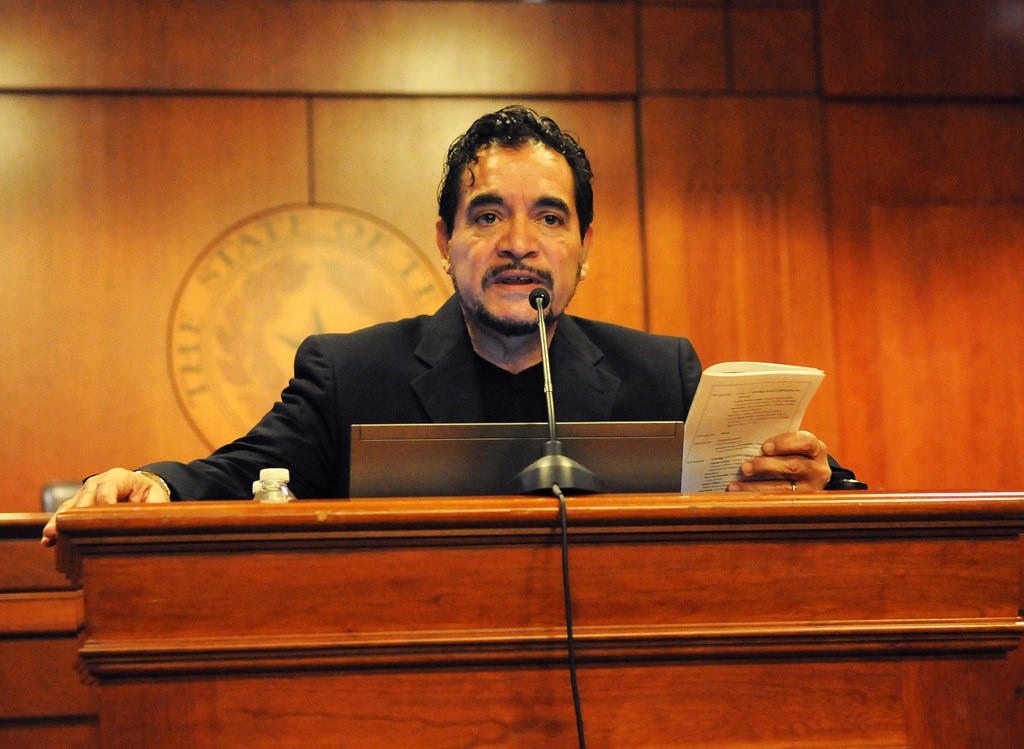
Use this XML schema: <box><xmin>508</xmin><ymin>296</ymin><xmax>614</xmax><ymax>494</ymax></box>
<box><xmin>501</xmin><ymin>287</ymin><xmax>605</xmax><ymax>496</ymax></box>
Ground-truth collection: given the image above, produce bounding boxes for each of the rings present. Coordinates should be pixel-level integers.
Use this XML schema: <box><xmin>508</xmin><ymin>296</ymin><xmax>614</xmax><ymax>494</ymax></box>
<box><xmin>791</xmin><ymin>480</ymin><xmax>796</xmax><ymax>491</ymax></box>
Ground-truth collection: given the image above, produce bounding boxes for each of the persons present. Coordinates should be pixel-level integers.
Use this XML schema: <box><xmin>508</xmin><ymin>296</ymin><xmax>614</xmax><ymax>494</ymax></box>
<box><xmin>39</xmin><ymin>106</ymin><xmax>858</xmax><ymax>553</ymax></box>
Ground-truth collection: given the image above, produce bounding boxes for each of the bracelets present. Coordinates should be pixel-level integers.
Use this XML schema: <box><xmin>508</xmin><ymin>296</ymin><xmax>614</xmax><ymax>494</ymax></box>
<box><xmin>136</xmin><ymin>470</ymin><xmax>170</xmax><ymax>495</ymax></box>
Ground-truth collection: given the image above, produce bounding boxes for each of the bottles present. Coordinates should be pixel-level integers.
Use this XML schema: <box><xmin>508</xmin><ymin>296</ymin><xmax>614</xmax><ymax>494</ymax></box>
<box><xmin>252</xmin><ymin>468</ymin><xmax>296</xmax><ymax>503</ymax></box>
